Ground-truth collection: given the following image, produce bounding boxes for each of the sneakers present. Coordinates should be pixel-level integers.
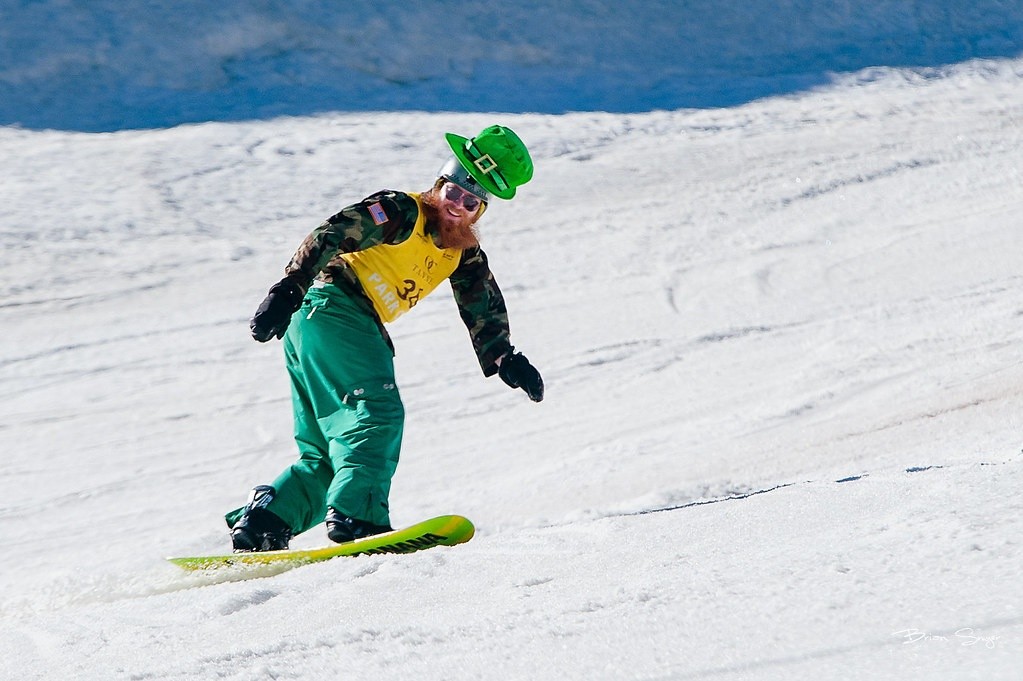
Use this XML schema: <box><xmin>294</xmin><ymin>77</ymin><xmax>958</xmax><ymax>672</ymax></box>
<box><xmin>325</xmin><ymin>505</ymin><xmax>396</xmax><ymax>543</ymax></box>
<box><xmin>230</xmin><ymin>510</ymin><xmax>298</xmax><ymax>552</ymax></box>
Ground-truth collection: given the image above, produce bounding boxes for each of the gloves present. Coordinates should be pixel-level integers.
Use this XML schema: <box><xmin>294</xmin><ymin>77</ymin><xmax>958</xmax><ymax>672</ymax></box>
<box><xmin>500</xmin><ymin>353</ymin><xmax>544</xmax><ymax>403</ymax></box>
<box><xmin>249</xmin><ymin>276</ymin><xmax>308</xmax><ymax>343</ymax></box>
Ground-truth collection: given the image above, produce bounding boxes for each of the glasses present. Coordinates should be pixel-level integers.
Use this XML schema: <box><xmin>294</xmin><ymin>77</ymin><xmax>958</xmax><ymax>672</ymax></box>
<box><xmin>442</xmin><ymin>181</ymin><xmax>482</xmax><ymax>213</ymax></box>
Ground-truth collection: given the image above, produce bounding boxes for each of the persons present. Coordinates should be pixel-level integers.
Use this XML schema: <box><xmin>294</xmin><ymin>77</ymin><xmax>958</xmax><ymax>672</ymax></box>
<box><xmin>226</xmin><ymin>154</ymin><xmax>544</xmax><ymax>549</ymax></box>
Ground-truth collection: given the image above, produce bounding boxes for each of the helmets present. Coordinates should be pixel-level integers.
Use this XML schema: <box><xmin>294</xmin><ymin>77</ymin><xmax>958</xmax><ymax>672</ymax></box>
<box><xmin>431</xmin><ymin>153</ymin><xmax>490</xmax><ymax>224</ymax></box>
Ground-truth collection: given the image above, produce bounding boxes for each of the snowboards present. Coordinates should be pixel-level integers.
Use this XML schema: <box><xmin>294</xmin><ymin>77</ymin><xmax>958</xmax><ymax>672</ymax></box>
<box><xmin>165</xmin><ymin>514</ymin><xmax>475</xmax><ymax>572</ymax></box>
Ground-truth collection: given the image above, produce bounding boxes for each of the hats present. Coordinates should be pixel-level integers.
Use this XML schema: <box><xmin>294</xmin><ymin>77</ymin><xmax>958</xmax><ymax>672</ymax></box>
<box><xmin>445</xmin><ymin>124</ymin><xmax>533</xmax><ymax>201</ymax></box>
